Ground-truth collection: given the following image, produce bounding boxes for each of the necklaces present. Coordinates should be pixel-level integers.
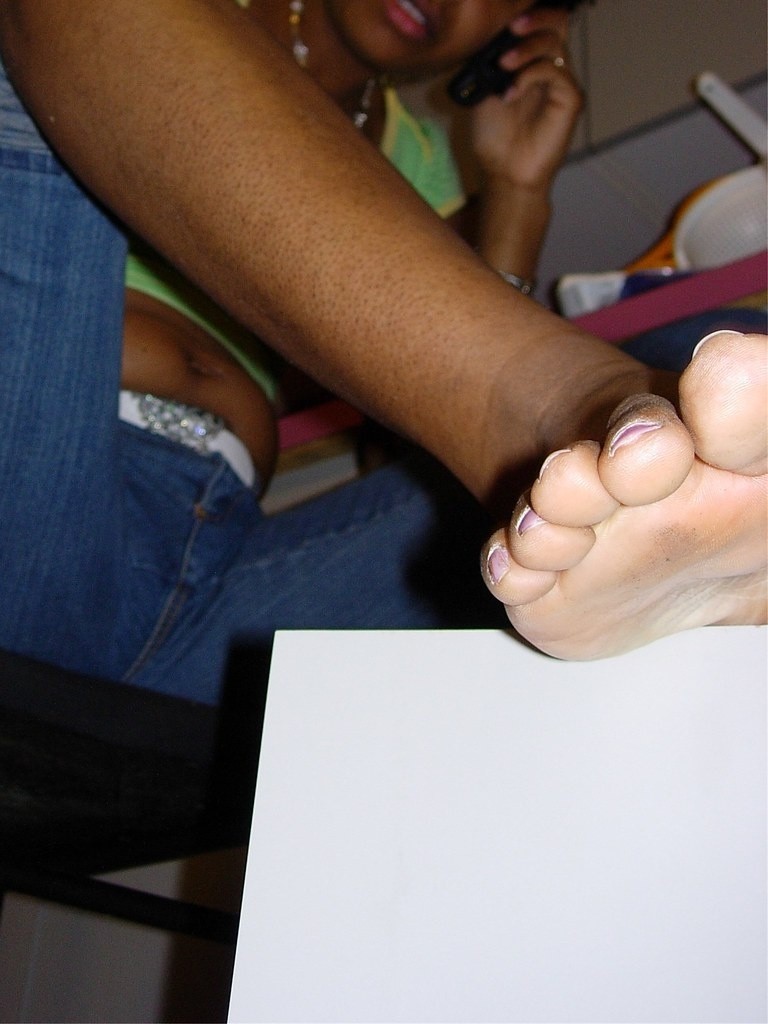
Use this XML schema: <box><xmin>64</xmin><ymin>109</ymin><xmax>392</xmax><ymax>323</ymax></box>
<box><xmin>287</xmin><ymin>0</ymin><xmax>376</xmax><ymax>130</ymax></box>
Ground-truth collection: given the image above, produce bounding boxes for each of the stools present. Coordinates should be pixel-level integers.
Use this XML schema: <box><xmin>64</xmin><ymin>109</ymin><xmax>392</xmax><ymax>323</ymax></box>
<box><xmin>0</xmin><ymin>634</ymin><xmax>264</xmax><ymax>941</ymax></box>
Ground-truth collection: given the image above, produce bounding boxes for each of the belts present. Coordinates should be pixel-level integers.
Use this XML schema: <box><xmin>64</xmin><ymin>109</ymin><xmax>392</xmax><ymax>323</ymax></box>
<box><xmin>115</xmin><ymin>391</ymin><xmax>256</xmax><ymax>490</ymax></box>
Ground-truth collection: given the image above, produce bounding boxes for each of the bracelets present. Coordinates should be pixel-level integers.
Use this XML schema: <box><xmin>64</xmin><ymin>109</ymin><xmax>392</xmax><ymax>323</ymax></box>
<box><xmin>498</xmin><ymin>272</ymin><xmax>531</xmax><ymax>294</ymax></box>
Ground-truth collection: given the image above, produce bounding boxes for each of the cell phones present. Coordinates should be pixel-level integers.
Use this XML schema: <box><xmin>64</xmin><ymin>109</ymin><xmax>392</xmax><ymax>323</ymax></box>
<box><xmin>445</xmin><ymin>28</ymin><xmax>528</xmax><ymax>110</ymax></box>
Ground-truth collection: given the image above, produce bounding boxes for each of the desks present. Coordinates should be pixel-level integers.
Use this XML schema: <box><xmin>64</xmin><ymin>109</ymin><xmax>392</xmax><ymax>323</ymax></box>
<box><xmin>227</xmin><ymin>625</ymin><xmax>765</xmax><ymax>1024</ymax></box>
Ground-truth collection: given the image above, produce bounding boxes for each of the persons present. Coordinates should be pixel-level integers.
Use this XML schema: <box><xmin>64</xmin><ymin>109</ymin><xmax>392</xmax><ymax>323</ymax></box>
<box><xmin>0</xmin><ymin>0</ymin><xmax>768</xmax><ymax>725</ymax></box>
